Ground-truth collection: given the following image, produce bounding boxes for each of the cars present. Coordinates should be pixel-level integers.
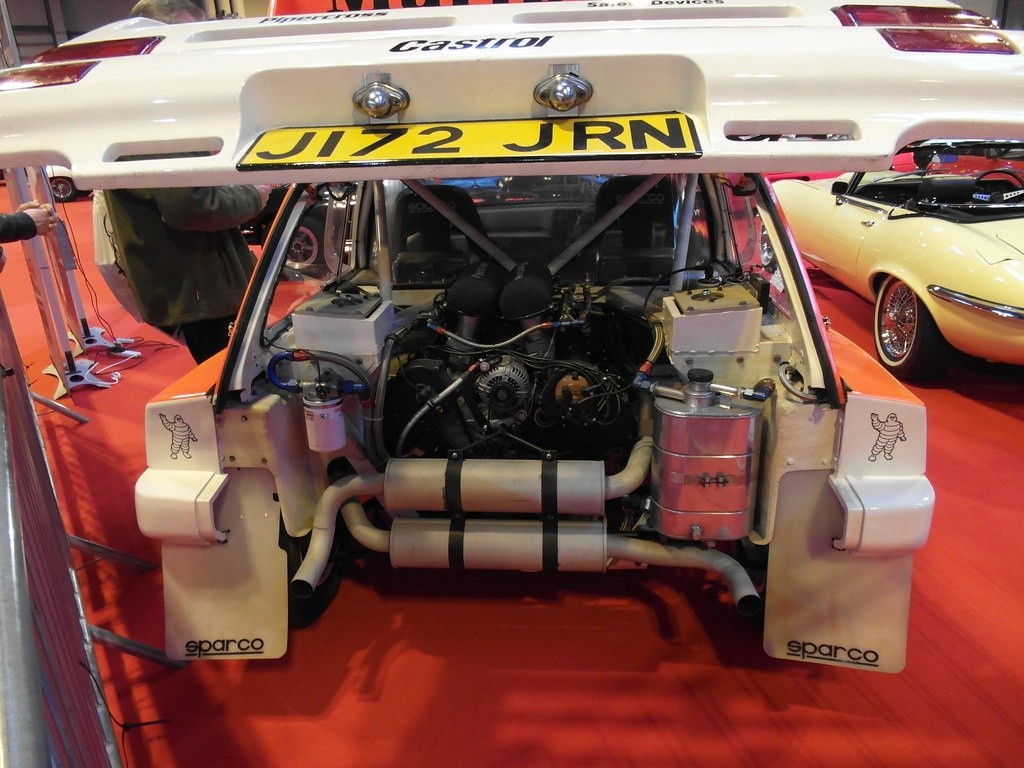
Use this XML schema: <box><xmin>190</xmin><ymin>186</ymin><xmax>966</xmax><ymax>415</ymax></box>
<box><xmin>768</xmin><ymin>138</ymin><xmax>1024</xmax><ymax>379</ymax></box>
<box><xmin>0</xmin><ymin>0</ymin><xmax>1024</xmax><ymax>679</ymax></box>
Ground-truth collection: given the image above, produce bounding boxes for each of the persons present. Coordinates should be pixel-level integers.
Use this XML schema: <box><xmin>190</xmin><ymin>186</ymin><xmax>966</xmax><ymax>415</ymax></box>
<box><xmin>102</xmin><ymin>0</ymin><xmax>276</xmax><ymax>366</ymax></box>
<box><xmin>0</xmin><ymin>198</ymin><xmax>60</xmax><ymax>245</ymax></box>
